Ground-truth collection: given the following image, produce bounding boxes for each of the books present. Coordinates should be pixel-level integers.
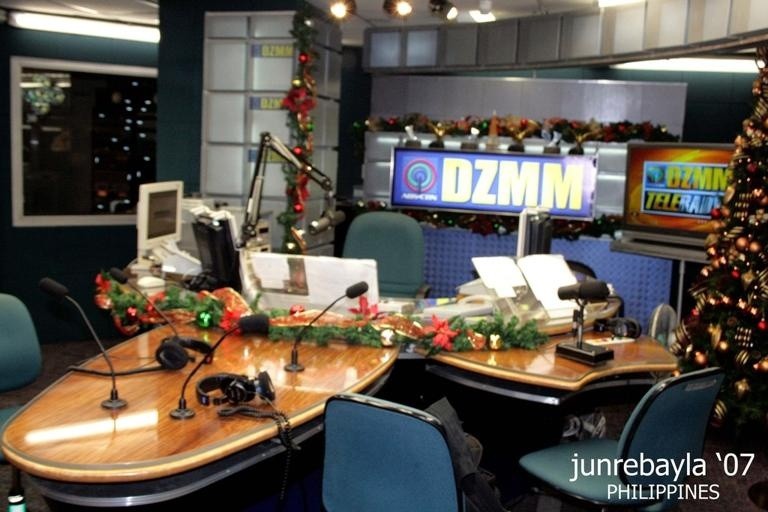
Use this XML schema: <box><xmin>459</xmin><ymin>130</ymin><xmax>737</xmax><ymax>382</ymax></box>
<box><xmin>456</xmin><ymin>249</ymin><xmax>587</xmax><ymax>339</ymax></box>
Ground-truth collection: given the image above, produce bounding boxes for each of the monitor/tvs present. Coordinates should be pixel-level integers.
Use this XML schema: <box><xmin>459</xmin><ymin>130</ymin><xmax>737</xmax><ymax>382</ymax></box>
<box><xmin>192</xmin><ymin>212</ymin><xmax>242</xmax><ymax>291</ymax></box>
<box><xmin>621</xmin><ymin>139</ymin><xmax>736</xmax><ymax>248</ymax></box>
<box><xmin>240</xmin><ymin>247</ymin><xmax>379</xmax><ymax>320</ymax></box>
<box><xmin>134</xmin><ymin>179</ymin><xmax>183</xmax><ymax>249</ymax></box>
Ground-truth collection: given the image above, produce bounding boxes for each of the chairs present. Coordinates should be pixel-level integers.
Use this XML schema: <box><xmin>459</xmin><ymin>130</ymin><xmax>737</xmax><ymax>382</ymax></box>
<box><xmin>344</xmin><ymin>212</ymin><xmax>430</xmax><ymax>300</ymax></box>
<box><xmin>320</xmin><ymin>393</ymin><xmax>474</xmax><ymax>511</ymax></box>
<box><xmin>0</xmin><ymin>294</ymin><xmax>43</xmax><ymax>491</ymax></box>
<box><xmin>520</xmin><ymin>367</ymin><xmax>724</xmax><ymax>512</ymax></box>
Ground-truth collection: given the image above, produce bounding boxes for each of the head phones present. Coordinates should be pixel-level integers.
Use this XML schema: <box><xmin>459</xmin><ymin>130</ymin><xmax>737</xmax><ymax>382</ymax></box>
<box><xmin>196</xmin><ymin>369</ymin><xmax>277</xmax><ymax>408</ymax></box>
<box><xmin>594</xmin><ymin>318</ymin><xmax>644</xmax><ymax>339</ymax></box>
<box><xmin>155</xmin><ymin>334</ymin><xmax>214</xmax><ymax>370</ymax></box>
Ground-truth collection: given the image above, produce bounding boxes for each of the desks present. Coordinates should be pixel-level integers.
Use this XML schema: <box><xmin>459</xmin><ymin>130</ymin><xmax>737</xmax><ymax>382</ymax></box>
<box><xmin>0</xmin><ymin>293</ymin><xmax>677</xmax><ymax>512</ymax></box>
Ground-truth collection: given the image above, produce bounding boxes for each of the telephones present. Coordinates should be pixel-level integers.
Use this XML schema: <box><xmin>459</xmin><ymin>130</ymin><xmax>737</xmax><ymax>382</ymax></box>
<box><xmin>422</xmin><ymin>296</ymin><xmax>495</xmax><ymax>321</ymax></box>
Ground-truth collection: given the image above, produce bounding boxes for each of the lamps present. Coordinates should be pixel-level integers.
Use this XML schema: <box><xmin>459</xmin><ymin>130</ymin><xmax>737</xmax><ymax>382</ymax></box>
<box><xmin>428</xmin><ymin>0</ymin><xmax>458</xmax><ymax>21</ymax></box>
<box><xmin>330</xmin><ymin>0</ymin><xmax>358</xmax><ymax>22</ymax></box>
<box><xmin>383</xmin><ymin>0</ymin><xmax>412</xmax><ymax>17</ymax></box>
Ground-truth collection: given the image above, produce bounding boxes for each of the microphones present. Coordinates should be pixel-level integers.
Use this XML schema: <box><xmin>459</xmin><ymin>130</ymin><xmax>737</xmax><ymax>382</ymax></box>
<box><xmin>38</xmin><ymin>278</ymin><xmax>128</xmax><ymax>409</ymax></box>
<box><xmin>170</xmin><ymin>313</ymin><xmax>270</xmax><ymax>420</ymax></box>
<box><xmin>109</xmin><ymin>268</ymin><xmax>182</xmax><ymax>346</ymax></box>
<box><xmin>308</xmin><ymin>210</ymin><xmax>347</xmax><ymax>236</ymax></box>
<box><xmin>283</xmin><ymin>282</ymin><xmax>369</xmax><ymax>372</ymax></box>
<box><xmin>558</xmin><ymin>279</ymin><xmax>610</xmax><ymax>303</ymax></box>
